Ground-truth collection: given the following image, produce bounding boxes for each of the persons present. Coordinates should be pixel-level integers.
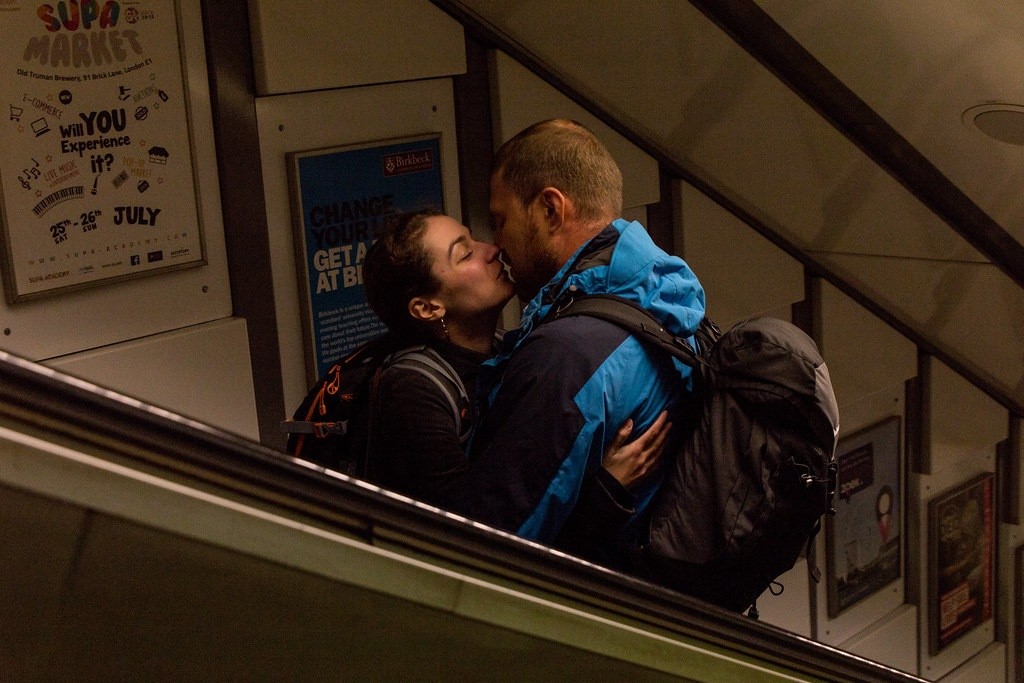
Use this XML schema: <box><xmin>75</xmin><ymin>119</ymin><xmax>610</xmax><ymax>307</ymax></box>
<box><xmin>354</xmin><ymin>212</ymin><xmax>673</xmax><ymax>558</ymax></box>
<box><xmin>463</xmin><ymin>119</ymin><xmax>706</xmax><ymax>577</ymax></box>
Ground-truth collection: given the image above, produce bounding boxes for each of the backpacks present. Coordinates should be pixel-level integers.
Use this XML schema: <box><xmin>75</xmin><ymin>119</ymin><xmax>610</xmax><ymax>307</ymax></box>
<box><xmin>537</xmin><ymin>296</ymin><xmax>841</xmax><ymax>612</ymax></box>
<box><xmin>287</xmin><ymin>333</ymin><xmax>474</xmax><ymax>480</ymax></box>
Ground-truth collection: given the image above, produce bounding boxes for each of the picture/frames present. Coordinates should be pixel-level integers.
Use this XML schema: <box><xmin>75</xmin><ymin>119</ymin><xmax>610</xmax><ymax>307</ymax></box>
<box><xmin>927</xmin><ymin>471</ymin><xmax>996</xmax><ymax>658</ymax></box>
<box><xmin>995</xmin><ymin>437</ymin><xmax>1009</xmax><ymax>639</ymax></box>
<box><xmin>824</xmin><ymin>414</ymin><xmax>904</xmax><ymax>621</ymax></box>
<box><xmin>0</xmin><ymin>0</ymin><xmax>207</xmax><ymax>307</ymax></box>
<box><xmin>285</xmin><ymin>132</ymin><xmax>448</xmax><ymax>391</ymax></box>
